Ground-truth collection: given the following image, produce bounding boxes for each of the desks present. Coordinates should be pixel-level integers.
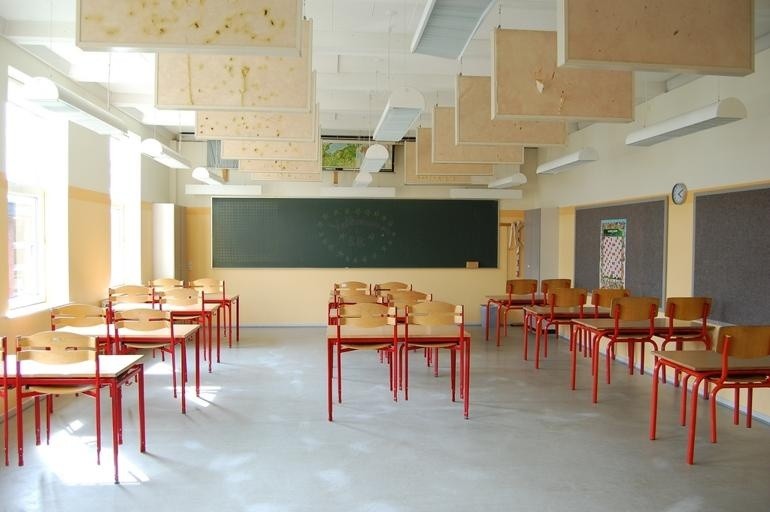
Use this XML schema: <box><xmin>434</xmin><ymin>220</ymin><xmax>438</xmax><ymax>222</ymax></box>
<box><xmin>0</xmin><ymin>355</ymin><xmax>145</xmax><ymax>484</ymax></box>
<box><xmin>327</xmin><ymin>326</ymin><xmax>472</xmax><ymax>421</ymax></box>
<box><xmin>145</xmin><ymin>296</ymin><xmax>239</xmax><ymax>349</ymax></box>
<box><xmin>650</xmin><ymin>349</ymin><xmax>770</xmax><ymax>465</ymax></box>
<box><xmin>568</xmin><ymin>317</ymin><xmax>711</xmax><ymax>403</ymax></box>
<box><xmin>46</xmin><ymin>320</ymin><xmax>203</xmax><ymax>417</ymax></box>
<box><xmin>104</xmin><ymin>301</ymin><xmax>221</xmax><ymax>372</ymax></box>
<box><xmin>525</xmin><ymin>305</ymin><xmax>623</xmax><ymax>371</ymax></box>
<box><xmin>484</xmin><ymin>294</ymin><xmax>573</xmax><ymax>348</ymax></box>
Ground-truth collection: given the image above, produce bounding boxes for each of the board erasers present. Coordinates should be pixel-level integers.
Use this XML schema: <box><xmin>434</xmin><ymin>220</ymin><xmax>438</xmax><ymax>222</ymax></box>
<box><xmin>466</xmin><ymin>261</ymin><xmax>479</xmax><ymax>268</ymax></box>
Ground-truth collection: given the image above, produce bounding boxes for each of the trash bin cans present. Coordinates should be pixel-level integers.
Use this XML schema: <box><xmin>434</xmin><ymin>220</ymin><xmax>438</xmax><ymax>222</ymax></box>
<box><xmin>479</xmin><ymin>304</ymin><xmax>498</xmax><ymax>329</ymax></box>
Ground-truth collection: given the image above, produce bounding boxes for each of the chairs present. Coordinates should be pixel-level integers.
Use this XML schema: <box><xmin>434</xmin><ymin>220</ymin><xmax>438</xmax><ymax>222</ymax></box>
<box><xmin>589</xmin><ymin>288</ymin><xmax>629</xmax><ymax>361</ymax></box>
<box><xmin>158</xmin><ymin>288</ymin><xmax>205</xmax><ymax>359</ymax></box>
<box><xmin>545</xmin><ymin>288</ymin><xmax>585</xmax><ymax>361</ymax></box>
<box><xmin>334</xmin><ymin>283</ymin><xmax>365</xmax><ymax>299</ymax></box>
<box><xmin>385</xmin><ymin>292</ymin><xmax>433</xmax><ymax>371</ymax></box>
<box><xmin>399</xmin><ymin>301</ymin><xmax>463</xmax><ymax>402</ymax></box>
<box><xmin>0</xmin><ymin>336</ymin><xmax>51</xmax><ymax>465</ymax></box>
<box><xmin>147</xmin><ymin>278</ymin><xmax>185</xmax><ymax>290</ymax></box>
<box><xmin>504</xmin><ymin>279</ymin><xmax>538</xmax><ymax>338</ymax></box>
<box><xmin>337</xmin><ymin>294</ymin><xmax>389</xmax><ymax>367</ymax></box>
<box><xmin>112</xmin><ymin>308</ymin><xmax>177</xmax><ymax>401</ymax></box>
<box><xmin>188</xmin><ymin>278</ymin><xmax>226</xmax><ymax>337</ymax></box>
<box><xmin>539</xmin><ymin>278</ymin><xmax>572</xmax><ymax>344</ymax></box>
<box><xmin>50</xmin><ymin>307</ymin><xmax>110</xmax><ymax>354</ymax></box>
<box><xmin>336</xmin><ymin>303</ymin><xmax>398</xmax><ymax>404</ymax></box>
<box><xmin>107</xmin><ymin>286</ymin><xmax>154</xmax><ymax>322</ymax></box>
<box><xmin>15</xmin><ymin>330</ymin><xmax>99</xmax><ymax>467</ymax></box>
<box><xmin>373</xmin><ymin>283</ymin><xmax>414</xmax><ymax>297</ymax></box>
<box><xmin>707</xmin><ymin>325</ymin><xmax>770</xmax><ymax>446</ymax></box>
<box><xmin>658</xmin><ymin>297</ymin><xmax>711</xmax><ymax>384</ymax></box>
<box><xmin>604</xmin><ymin>297</ymin><xmax>659</xmax><ymax>384</ymax></box>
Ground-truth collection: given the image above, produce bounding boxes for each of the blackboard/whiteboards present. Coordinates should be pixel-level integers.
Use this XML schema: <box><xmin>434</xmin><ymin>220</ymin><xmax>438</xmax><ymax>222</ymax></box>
<box><xmin>211</xmin><ymin>196</ymin><xmax>500</xmax><ymax>269</ymax></box>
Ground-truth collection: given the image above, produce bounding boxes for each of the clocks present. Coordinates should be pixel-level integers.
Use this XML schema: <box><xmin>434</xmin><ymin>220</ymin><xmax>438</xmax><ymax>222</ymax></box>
<box><xmin>672</xmin><ymin>183</ymin><xmax>687</xmax><ymax>207</ymax></box>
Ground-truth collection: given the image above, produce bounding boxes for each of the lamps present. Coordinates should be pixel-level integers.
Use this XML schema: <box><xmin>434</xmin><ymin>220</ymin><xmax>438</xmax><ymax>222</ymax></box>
<box><xmin>353</xmin><ymin>176</ymin><xmax>372</xmax><ymax>187</ymax></box>
<box><xmin>536</xmin><ymin>151</ymin><xmax>596</xmax><ymax>173</ymax></box>
<box><xmin>23</xmin><ymin>76</ymin><xmax>125</xmax><ymax>136</ymax></box>
<box><xmin>193</xmin><ymin>166</ymin><xmax>226</xmax><ymax>187</ymax></box>
<box><xmin>372</xmin><ymin>98</ymin><xmax>424</xmax><ymax>142</ymax></box>
<box><xmin>145</xmin><ymin>142</ymin><xmax>188</xmax><ymax>171</ymax></box>
<box><xmin>624</xmin><ymin>97</ymin><xmax>747</xmax><ymax>147</ymax></box>
<box><xmin>487</xmin><ymin>174</ymin><xmax>528</xmax><ymax>191</ymax></box>
<box><xmin>359</xmin><ymin>145</ymin><xmax>389</xmax><ymax>174</ymax></box>
<box><xmin>408</xmin><ymin>0</ymin><xmax>496</xmax><ymax>65</ymax></box>
